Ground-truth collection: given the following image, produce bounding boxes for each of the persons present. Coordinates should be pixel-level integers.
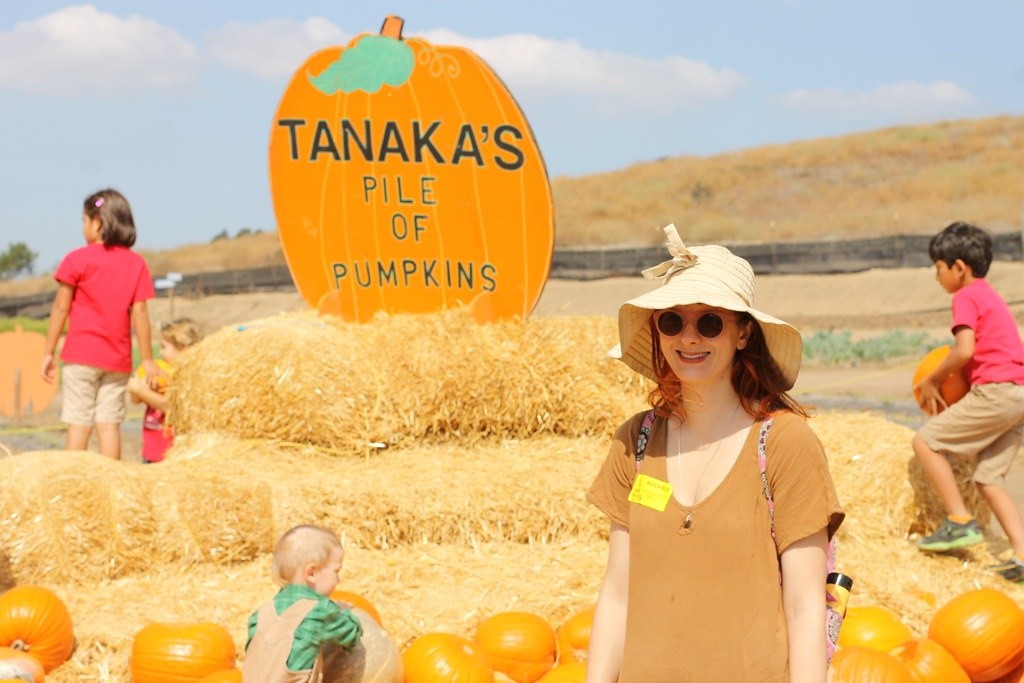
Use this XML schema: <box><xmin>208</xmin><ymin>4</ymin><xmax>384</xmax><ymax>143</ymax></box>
<box><xmin>912</xmin><ymin>220</ymin><xmax>1024</xmax><ymax>582</ymax></box>
<box><xmin>39</xmin><ymin>188</ymin><xmax>160</xmax><ymax>461</ymax></box>
<box><xmin>131</xmin><ymin>318</ymin><xmax>205</xmax><ymax>465</ymax></box>
<box><xmin>586</xmin><ymin>302</ymin><xmax>846</xmax><ymax>683</ymax></box>
<box><xmin>243</xmin><ymin>524</ymin><xmax>363</xmax><ymax>683</ymax></box>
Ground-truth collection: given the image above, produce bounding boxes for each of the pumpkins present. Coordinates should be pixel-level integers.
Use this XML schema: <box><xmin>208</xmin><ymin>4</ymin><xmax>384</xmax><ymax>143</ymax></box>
<box><xmin>0</xmin><ymin>584</ymin><xmax>74</xmax><ymax>683</ymax></box>
<box><xmin>912</xmin><ymin>345</ymin><xmax>971</xmax><ymax>415</ymax></box>
<box><xmin>131</xmin><ymin>359</ymin><xmax>170</xmax><ymax>403</ymax></box>
<box><xmin>127</xmin><ymin>587</ymin><xmax>1024</xmax><ymax>683</ymax></box>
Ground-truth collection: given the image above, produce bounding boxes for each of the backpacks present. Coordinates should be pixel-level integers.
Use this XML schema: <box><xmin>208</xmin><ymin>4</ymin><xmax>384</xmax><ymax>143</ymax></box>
<box><xmin>633</xmin><ymin>409</ymin><xmax>853</xmax><ymax>672</ymax></box>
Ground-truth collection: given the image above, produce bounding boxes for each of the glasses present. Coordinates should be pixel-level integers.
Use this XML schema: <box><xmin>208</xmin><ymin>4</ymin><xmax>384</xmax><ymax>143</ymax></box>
<box><xmin>652</xmin><ymin>307</ymin><xmax>741</xmax><ymax>341</ymax></box>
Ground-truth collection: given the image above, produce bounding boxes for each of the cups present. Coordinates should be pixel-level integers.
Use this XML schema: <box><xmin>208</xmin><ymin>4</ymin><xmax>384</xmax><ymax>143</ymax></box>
<box><xmin>826</xmin><ymin>572</ymin><xmax>853</xmax><ymax>618</ymax></box>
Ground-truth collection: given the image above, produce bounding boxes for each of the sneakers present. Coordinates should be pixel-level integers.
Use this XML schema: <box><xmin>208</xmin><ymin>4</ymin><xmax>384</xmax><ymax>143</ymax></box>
<box><xmin>918</xmin><ymin>514</ymin><xmax>984</xmax><ymax>553</ymax></box>
<box><xmin>987</xmin><ymin>557</ymin><xmax>1024</xmax><ymax>586</ymax></box>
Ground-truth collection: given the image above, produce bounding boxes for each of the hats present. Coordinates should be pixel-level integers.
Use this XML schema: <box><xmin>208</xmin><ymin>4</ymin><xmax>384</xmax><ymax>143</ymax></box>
<box><xmin>607</xmin><ymin>222</ymin><xmax>802</xmax><ymax>390</ymax></box>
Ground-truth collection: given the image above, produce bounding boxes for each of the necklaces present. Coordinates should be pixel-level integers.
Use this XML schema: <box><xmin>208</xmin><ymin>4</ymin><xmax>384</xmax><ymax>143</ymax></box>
<box><xmin>678</xmin><ymin>399</ymin><xmax>742</xmax><ymax>537</ymax></box>
